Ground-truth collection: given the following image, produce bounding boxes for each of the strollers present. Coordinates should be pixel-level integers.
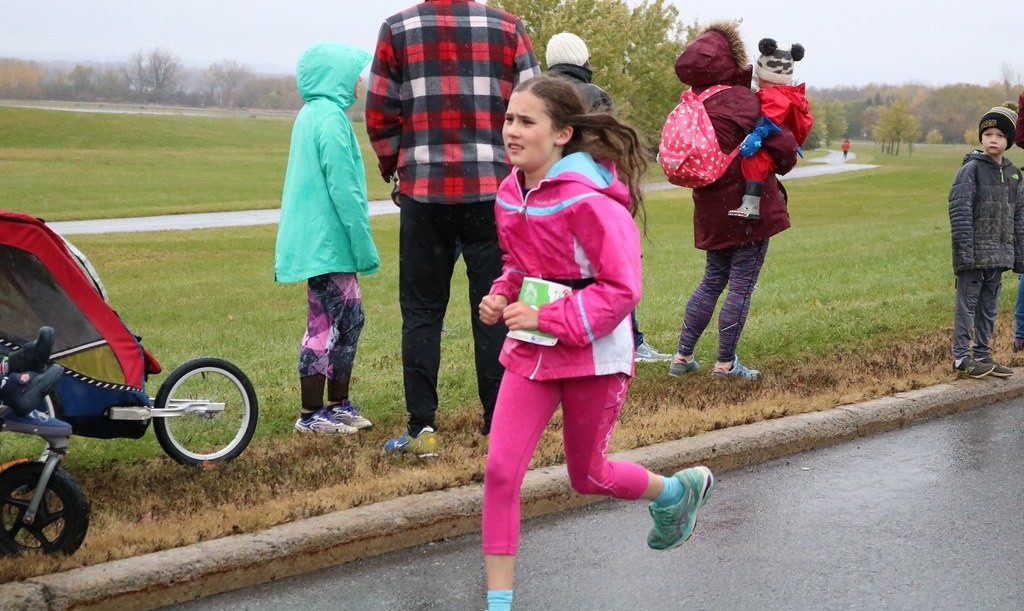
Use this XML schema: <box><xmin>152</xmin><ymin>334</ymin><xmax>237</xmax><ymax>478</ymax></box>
<box><xmin>0</xmin><ymin>210</ymin><xmax>259</xmax><ymax>566</ymax></box>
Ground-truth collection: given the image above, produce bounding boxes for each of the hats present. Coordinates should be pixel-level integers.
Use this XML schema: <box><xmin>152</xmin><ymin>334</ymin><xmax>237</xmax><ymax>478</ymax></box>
<box><xmin>755</xmin><ymin>38</ymin><xmax>804</xmax><ymax>84</ymax></box>
<box><xmin>546</xmin><ymin>33</ymin><xmax>589</xmax><ymax>66</ymax></box>
<box><xmin>979</xmin><ymin>101</ymin><xmax>1019</xmax><ymax>150</ymax></box>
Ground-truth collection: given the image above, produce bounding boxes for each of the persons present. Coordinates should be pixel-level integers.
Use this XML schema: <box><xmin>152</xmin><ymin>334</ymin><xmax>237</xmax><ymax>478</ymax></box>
<box><xmin>479</xmin><ymin>76</ymin><xmax>714</xmax><ymax>611</ymax></box>
<box><xmin>1013</xmin><ymin>91</ymin><xmax>1024</xmax><ymax>353</ymax></box>
<box><xmin>669</xmin><ymin>23</ymin><xmax>799</xmax><ymax>381</ymax></box>
<box><xmin>948</xmin><ymin>101</ymin><xmax>1024</xmax><ymax>378</ymax></box>
<box><xmin>545</xmin><ymin>32</ymin><xmax>673</xmax><ymax>361</ymax></box>
<box><xmin>728</xmin><ymin>38</ymin><xmax>813</xmax><ymax>221</ymax></box>
<box><xmin>366</xmin><ymin>1</ymin><xmax>542</xmax><ymax>461</ymax></box>
<box><xmin>274</xmin><ymin>42</ymin><xmax>383</xmax><ymax>434</ymax></box>
<box><xmin>843</xmin><ymin>141</ymin><xmax>850</xmax><ymax>158</ymax></box>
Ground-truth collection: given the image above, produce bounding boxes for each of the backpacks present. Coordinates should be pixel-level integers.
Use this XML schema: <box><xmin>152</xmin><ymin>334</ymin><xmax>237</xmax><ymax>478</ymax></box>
<box><xmin>656</xmin><ymin>84</ymin><xmax>739</xmax><ymax>189</ymax></box>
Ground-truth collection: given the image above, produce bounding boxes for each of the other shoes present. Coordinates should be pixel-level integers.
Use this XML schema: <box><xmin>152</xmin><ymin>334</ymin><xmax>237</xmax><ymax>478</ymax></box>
<box><xmin>1014</xmin><ymin>339</ymin><xmax>1024</xmax><ymax>352</ymax></box>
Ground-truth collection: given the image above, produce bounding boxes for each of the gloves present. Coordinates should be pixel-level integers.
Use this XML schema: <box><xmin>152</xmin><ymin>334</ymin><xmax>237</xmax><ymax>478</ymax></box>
<box><xmin>740</xmin><ymin>131</ymin><xmax>763</xmax><ymax>157</ymax></box>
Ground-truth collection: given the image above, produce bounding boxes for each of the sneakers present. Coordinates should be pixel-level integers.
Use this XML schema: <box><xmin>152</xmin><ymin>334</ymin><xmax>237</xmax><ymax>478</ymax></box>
<box><xmin>327</xmin><ymin>399</ymin><xmax>372</xmax><ymax>429</ymax></box>
<box><xmin>668</xmin><ymin>353</ymin><xmax>699</xmax><ymax>377</ymax></box>
<box><xmin>381</xmin><ymin>425</ymin><xmax>440</xmax><ymax>457</ymax></box>
<box><xmin>294</xmin><ymin>407</ymin><xmax>358</xmax><ymax>434</ymax></box>
<box><xmin>646</xmin><ymin>466</ymin><xmax>714</xmax><ymax>550</ymax></box>
<box><xmin>953</xmin><ymin>357</ymin><xmax>995</xmax><ymax>378</ymax></box>
<box><xmin>6</xmin><ymin>327</ymin><xmax>55</xmax><ymax>374</ymax></box>
<box><xmin>973</xmin><ymin>357</ymin><xmax>1014</xmax><ymax>376</ymax></box>
<box><xmin>634</xmin><ymin>338</ymin><xmax>672</xmax><ymax>362</ymax></box>
<box><xmin>712</xmin><ymin>354</ymin><xmax>761</xmax><ymax>380</ymax></box>
<box><xmin>0</xmin><ymin>364</ymin><xmax>64</xmax><ymax>415</ymax></box>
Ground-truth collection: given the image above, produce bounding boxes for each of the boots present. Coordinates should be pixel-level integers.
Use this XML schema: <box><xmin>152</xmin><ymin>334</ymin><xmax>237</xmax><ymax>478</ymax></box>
<box><xmin>728</xmin><ymin>195</ymin><xmax>760</xmax><ymax>220</ymax></box>
